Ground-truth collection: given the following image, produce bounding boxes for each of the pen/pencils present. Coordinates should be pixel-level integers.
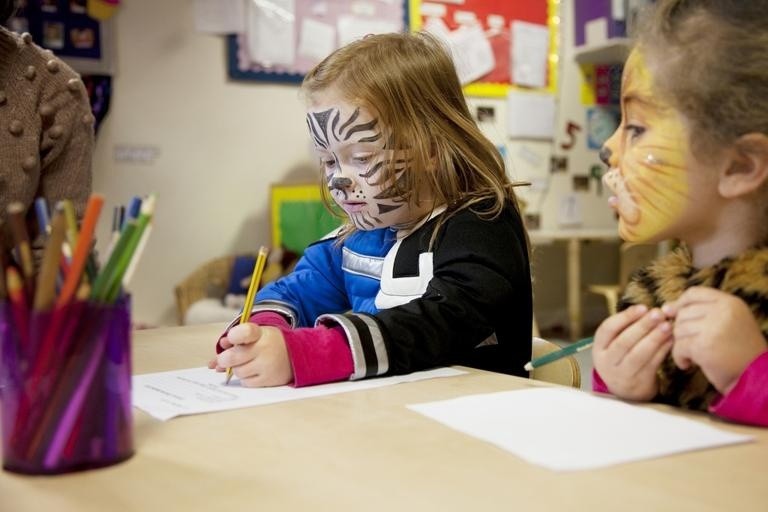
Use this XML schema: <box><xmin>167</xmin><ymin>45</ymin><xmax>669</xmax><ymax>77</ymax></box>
<box><xmin>525</xmin><ymin>335</ymin><xmax>596</xmax><ymax>372</ymax></box>
<box><xmin>223</xmin><ymin>245</ymin><xmax>272</xmax><ymax>388</ymax></box>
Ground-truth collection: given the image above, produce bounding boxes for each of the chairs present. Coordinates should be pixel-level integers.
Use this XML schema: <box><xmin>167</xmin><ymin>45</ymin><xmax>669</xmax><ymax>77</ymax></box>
<box><xmin>588</xmin><ymin>238</ymin><xmax>658</xmax><ymax>317</ymax></box>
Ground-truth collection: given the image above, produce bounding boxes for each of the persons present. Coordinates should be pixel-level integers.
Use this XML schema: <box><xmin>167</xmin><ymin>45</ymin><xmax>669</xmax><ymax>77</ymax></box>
<box><xmin>590</xmin><ymin>2</ymin><xmax>767</xmax><ymax>431</ymax></box>
<box><xmin>1</xmin><ymin>28</ymin><xmax>95</xmax><ymax>292</ymax></box>
<box><xmin>203</xmin><ymin>29</ymin><xmax>531</xmax><ymax>385</ymax></box>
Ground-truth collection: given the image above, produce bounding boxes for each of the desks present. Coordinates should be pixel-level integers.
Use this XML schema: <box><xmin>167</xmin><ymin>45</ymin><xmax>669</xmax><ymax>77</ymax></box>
<box><xmin>528</xmin><ymin>226</ymin><xmax>626</xmax><ymax>338</ymax></box>
<box><xmin>2</xmin><ymin>323</ymin><xmax>768</xmax><ymax>512</ymax></box>
